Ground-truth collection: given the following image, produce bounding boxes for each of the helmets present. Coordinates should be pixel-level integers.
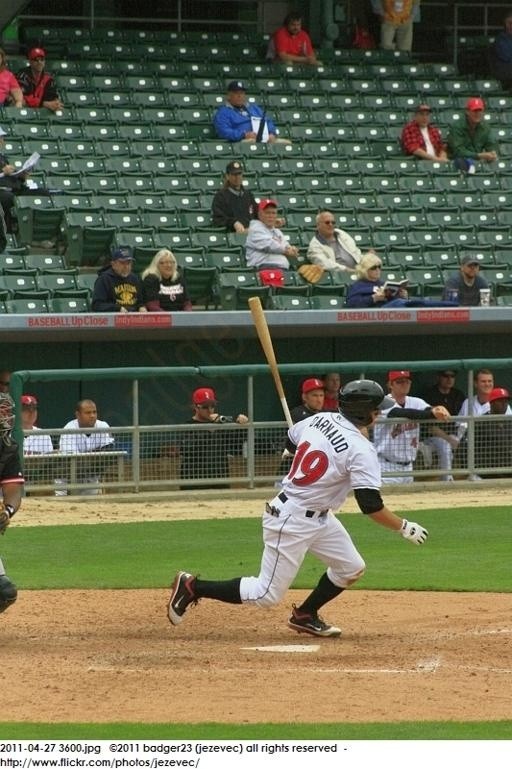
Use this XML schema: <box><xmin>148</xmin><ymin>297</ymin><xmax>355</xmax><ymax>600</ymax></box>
<box><xmin>338</xmin><ymin>379</ymin><xmax>384</xmax><ymax>426</ymax></box>
<box><xmin>489</xmin><ymin>387</ymin><xmax>509</xmax><ymax>403</ymax></box>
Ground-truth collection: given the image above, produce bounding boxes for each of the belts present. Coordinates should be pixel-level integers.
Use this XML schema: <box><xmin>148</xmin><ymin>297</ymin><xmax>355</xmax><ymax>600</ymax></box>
<box><xmin>380</xmin><ymin>452</ymin><xmax>411</xmax><ymax>465</ymax></box>
<box><xmin>278</xmin><ymin>491</ymin><xmax>329</xmax><ymax>519</ymax></box>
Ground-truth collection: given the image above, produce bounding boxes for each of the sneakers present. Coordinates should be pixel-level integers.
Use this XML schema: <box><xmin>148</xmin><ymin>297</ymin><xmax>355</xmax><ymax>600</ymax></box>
<box><xmin>288</xmin><ymin>602</ymin><xmax>342</xmax><ymax>637</ymax></box>
<box><xmin>167</xmin><ymin>571</ymin><xmax>196</xmax><ymax>625</ymax></box>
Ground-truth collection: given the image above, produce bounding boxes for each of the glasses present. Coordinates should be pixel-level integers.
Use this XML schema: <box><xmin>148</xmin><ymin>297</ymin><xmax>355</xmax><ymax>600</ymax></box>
<box><xmin>396</xmin><ymin>378</ymin><xmax>412</xmax><ymax>384</ymax></box>
<box><xmin>197</xmin><ymin>402</ymin><xmax>217</xmax><ymax>409</ymax></box>
<box><xmin>370</xmin><ymin>265</ymin><xmax>381</xmax><ymax>270</ymax></box>
<box><xmin>442</xmin><ymin>374</ymin><xmax>455</xmax><ymax>378</ymax></box>
<box><xmin>325</xmin><ymin>221</ymin><xmax>335</xmax><ymax>224</ymax></box>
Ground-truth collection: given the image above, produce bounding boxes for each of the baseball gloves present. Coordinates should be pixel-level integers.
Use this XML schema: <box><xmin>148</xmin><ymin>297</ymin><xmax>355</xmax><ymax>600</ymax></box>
<box><xmin>0</xmin><ymin>500</ymin><xmax>11</xmax><ymax>533</ymax></box>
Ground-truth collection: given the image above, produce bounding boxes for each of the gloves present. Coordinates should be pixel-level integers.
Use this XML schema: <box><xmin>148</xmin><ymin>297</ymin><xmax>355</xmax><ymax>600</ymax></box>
<box><xmin>282</xmin><ymin>448</ymin><xmax>295</xmax><ymax>460</ymax></box>
<box><xmin>399</xmin><ymin>519</ymin><xmax>428</xmax><ymax>545</ymax></box>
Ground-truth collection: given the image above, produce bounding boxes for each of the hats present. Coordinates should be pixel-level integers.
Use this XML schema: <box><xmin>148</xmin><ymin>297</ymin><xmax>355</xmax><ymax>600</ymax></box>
<box><xmin>112</xmin><ymin>247</ymin><xmax>136</xmax><ymax>262</ymax></box>
<box><xmin>303</xmin><ymin>378</ymin><xmax>324</xmax><ymax>393</ymax></box>
<box><xmin>259</xmin><ymin>199</ymin><xmax>277</xmax><ymax>209</ymax></box>
<box><xmin>193</xmin><ymin>387</ymin><xmax>216</xmax><ymax>404</ymax></box>
<box><xmin>228</xmin><ymin>81</ymin><xmax>248</xmax><ymax>91</ymax></box>
<box><xmin>29</xmin><ymin>48</ymin><xmax>45</xmax><ymax>58</ymax></box>
<box><xmin>226</xmin><ymin>160</ymin><xmax>244</xmax><ymax>174</ymax></box>
<box><xmin>21</xmin><ymin>394</ymin><xmax>39</xmax><ymax>409</ymax></box>
<box><xmin>415</xmin><ymin>104</ymin><xmax>431</xmax><ymax>113</ymax></box>
<box><xmin>259</xmin><ymin>270</ymin><xmax>283</xmax><ymax>285</ymax></box>
<box><xmin>389</xmin><ymin>370</ymin><xmax>411</xmax><ymax>380</ymax></box>
<box><xmin>462</xmin><ymin>256</ymin><xmax>480</xmax><ymax>266</ymax></box>
<box><xmin>467</xmin><ymin>97</ymin><xmax>485</xmax><ymax>111</ymax></box>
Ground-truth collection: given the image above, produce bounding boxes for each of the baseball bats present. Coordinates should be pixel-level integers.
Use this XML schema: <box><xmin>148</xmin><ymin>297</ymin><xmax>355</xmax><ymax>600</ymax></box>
<box><xmin>247</xmin><ymin>297</ymin><xmax>293</xmax><ymax>426</ymax></box>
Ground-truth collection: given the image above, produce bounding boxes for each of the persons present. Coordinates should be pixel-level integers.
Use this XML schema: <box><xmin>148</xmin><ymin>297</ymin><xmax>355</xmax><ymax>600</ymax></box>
<box><xmin>495</xmin><ymin>16</ymin><xmax>512</xmax><ymax>64</ymax></box>
<box><xmin>0</xmin><ymin>359</ymin><xmax>26</xmax><ymax>613</ymax></box>
<box><xmin>401</xmin><ymin>104</ymin><xmax>449</xmax><ymax>161</ymax></box>
<box><xmin>0</xmin><ymin>125</ymin><xmax>17</xmax><ymax>255</ymax></box>
<box><xmin>55</xmin><ymin>398</ymin><xmax>115</xmax><ymax>496</ymax></box>
<box><xmin>7</xmin><ymin>47</ymin><xmax>64</xmax><ymax>112</ymax></box>
<box><xmin>380</xmin><ymin>0</ymin><xmax>417</xmax><ymax>53</ymax></box>
<box><xmin>91</xmin><ymin>159</ymin><xmax>498</xmax><ymax>312</ymax></box>
<box><xmin>265</xmin><ymin>12</ymin><xmax>322</xmax><ymax>65</ymax></box>
<box><xmin>167</xmin><ymin>379</ymin><xmax>429</xmax><ymax>638</ymax></box>
<box><xmin>273</xmin><ymin>367</ymin><xmax>512</xmax><ymax>488</ymax></box>
<box><xmin>213</xmin><ymin>81</ymin><xmax>292</xmax><ymax>144</ymax></box>
<box><xmin>448</xmin><ymin>96</ymin><xmax>498</xmax><ymax>173</ymax></box>
<box><xmin>179</xmin><ymin>387</ymin><xmax>248</xmax><ymax>490</ymax></box>
<box><xmin>0</xmin><ymin>47</ymin><xmax>23</xmax><ymax>107</ymax></box>
<box><xmin>21</xmin><ymin>393</ymin><xmax>54</xmax><ymax>497</ymax></box>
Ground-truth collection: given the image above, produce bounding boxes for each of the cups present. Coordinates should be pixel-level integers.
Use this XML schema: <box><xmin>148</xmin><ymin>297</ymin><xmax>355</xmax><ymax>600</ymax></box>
<box><xmin>480</xmin><ymin>288</ymin><xmax>491</xmax><ymax>307</ymax></box>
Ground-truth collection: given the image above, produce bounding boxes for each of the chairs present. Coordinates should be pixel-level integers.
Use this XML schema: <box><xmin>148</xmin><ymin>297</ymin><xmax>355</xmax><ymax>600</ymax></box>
<box><xmin>0</xmin><ymin>28</ymin><xmax>512</xmax><ymax>322</ymax></box>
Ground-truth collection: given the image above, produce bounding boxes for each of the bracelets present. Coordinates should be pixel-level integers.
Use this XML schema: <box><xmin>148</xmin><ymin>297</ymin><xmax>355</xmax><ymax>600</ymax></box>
<box><xmin>5</xmin><ymin>504</ymin><xmax>15</xmax><ymax>518</ymax></box>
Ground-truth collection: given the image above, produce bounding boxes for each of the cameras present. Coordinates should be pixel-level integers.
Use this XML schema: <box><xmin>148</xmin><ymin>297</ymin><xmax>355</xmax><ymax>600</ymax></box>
<box><xmin>219</xmin><ymin>415</ymin><xmax>233</xmax><ymax>423</ymax></box>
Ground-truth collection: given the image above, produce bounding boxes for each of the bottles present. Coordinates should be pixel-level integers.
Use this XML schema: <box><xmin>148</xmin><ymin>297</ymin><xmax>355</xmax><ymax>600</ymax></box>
<box><xmin>242</xmin><ymin>441</ymin><xmax>247</xmax><ymax>459</ymax></box>
